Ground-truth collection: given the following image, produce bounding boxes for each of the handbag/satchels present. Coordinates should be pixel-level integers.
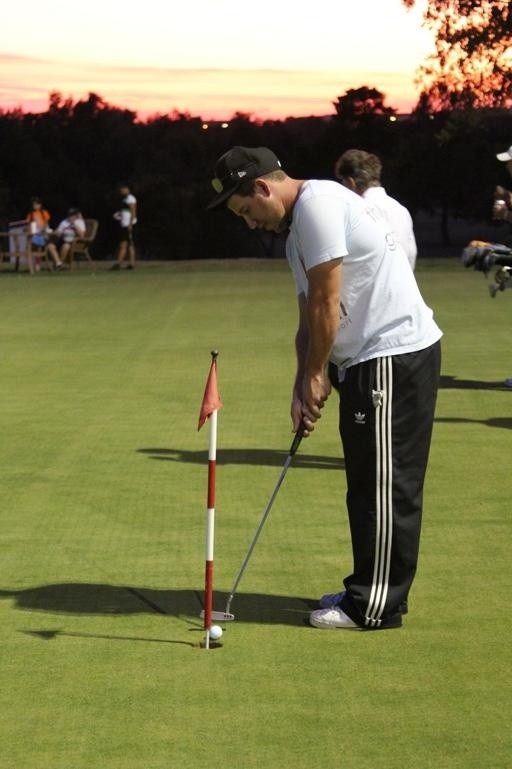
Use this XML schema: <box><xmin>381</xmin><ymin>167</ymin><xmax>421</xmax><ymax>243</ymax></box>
<box><xmin>32</xmin><ymin>235</ymin><xmax>47</xmax><ymax>246</ymax></box>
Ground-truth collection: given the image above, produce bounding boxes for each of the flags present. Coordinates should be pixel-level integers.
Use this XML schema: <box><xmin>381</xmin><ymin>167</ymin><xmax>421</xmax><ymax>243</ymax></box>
<box><xmin>194</xmin><ymin>360</ymin><xmax>224</xmax><ymax>430</ymax></box>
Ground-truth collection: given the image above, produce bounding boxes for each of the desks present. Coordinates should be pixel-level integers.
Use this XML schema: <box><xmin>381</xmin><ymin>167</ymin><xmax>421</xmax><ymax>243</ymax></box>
<box><xmin>0</xmin><ymin>231</ymin><xmax>54</xmax><ymax>276</ymax></box>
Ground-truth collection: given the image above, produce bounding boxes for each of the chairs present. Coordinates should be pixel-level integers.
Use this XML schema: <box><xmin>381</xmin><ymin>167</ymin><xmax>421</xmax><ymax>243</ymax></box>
<box><xmin>54</xmin><ymin>218</ymin><xmax>99</xmax><ymax>275</ymax></box>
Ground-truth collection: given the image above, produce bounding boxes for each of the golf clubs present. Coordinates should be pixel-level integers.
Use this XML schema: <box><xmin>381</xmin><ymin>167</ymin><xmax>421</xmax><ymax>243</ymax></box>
<box><xmin>463</xmin><ymin>241</ymin><xmax>512</xmax><ymax>298</ymax></box>
<box><xmin>200</xmin><ymin>415</ymin><xmax>305</xmax><ymax>621</ymax></box>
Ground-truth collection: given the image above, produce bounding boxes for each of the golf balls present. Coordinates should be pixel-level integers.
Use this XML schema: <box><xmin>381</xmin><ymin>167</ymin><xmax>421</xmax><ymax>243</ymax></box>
<box><xmin>209</xmin><ymin>625</ymin><xmax>222</xmax><ymax>639</ymax></box>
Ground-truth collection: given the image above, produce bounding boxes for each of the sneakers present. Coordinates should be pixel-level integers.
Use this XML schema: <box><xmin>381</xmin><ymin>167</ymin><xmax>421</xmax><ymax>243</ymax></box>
<box><xmin>308</xmin><ymin>592</ymin><xmax>408</xmax><ymax>628</ymax></box>
<box><xmin>110</xmin><ymin>264</ymin><xmax>133</xmax><ymax>270</ymax></box>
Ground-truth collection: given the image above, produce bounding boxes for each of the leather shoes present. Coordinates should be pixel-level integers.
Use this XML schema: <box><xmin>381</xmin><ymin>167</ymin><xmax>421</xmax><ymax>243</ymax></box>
<box><xmin>55</xmin><ymin>264</ymin><xmax>68</xmax><ymax>270</ymax></box>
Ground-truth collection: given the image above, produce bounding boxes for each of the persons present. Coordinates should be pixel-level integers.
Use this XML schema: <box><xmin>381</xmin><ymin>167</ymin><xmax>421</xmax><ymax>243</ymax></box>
<box><xmin>335</xmin><ymin>150</ymin><xmax>418</xmax><ymax>272</ymax></box>
<box><xmin>462</xmin><ymin>144</ymin><xmax>512</xmax><ymax>389</ymax></box>
<box><xmin>208</xmin><ymin>148</ymin><xmax>444</xmax><ymax>629</ymax></box>
<box><xmin>110</xmin><ymin>182</ymin><xmax>138</xmax><ymax>270</ymax></box>
<box><xmin>27</xmin><ymin>198</ymin><xmax>87</xmax><ymax>271</ymax></box>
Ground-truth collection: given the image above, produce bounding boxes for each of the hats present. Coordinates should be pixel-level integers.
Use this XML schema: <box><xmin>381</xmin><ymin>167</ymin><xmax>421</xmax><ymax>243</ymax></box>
<box><xmin>206</xmin><ymin>146</ymin><xmax>284</xmax><ymax>214</ymax></box>
<box><xmin>31</xmin><ymin>196</ymin><xmax>40</xmax><ymax>202</ymax></box>
<box><xmin>68</xmin><ymin>208</ymin><xmax>79</xmax><ymax>214</ymax></box>
<box><xmin>497</xmin><ymin>147</ymin><xmax>512</xmax><ymax>162</ymax></box>
